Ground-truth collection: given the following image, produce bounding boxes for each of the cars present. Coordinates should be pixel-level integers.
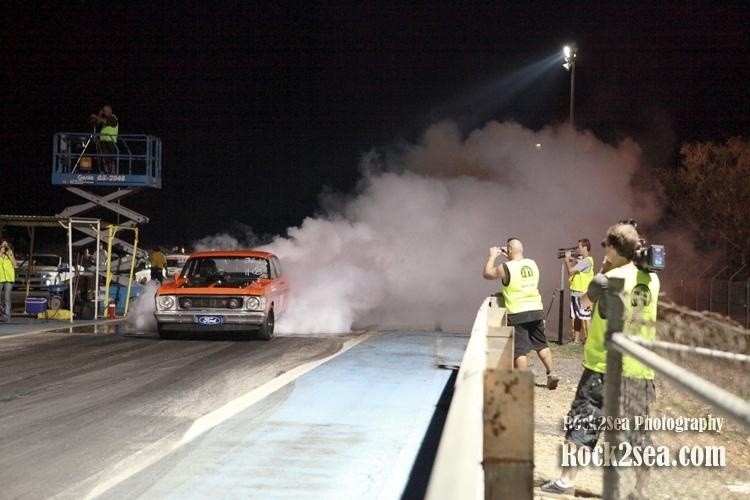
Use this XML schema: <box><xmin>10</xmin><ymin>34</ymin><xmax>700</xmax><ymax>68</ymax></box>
<box><xmin>1</xmin><ymin>247</ymin><xmax>296</xmax><ymax>341</ymax></box>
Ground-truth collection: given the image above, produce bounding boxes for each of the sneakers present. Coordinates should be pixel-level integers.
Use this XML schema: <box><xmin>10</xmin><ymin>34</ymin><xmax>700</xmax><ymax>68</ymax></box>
<box><xmin>624</xmin><ymin>491</ymin><xmax>649</xmax><ymax>500</ymax></box>
<box><xmin>547</xmin><ymin>370</ymin><xmax>560</xmax><ymax>389</ymax></box>
<box><xmin>567</xmin><ymin>341</ymin><xmax>583</xmax><ymax>345</ymax></box>
<box><xmin>541</xmin><ymin>479</ymin><xmax>575</xmax><ymax>496</ymax></box>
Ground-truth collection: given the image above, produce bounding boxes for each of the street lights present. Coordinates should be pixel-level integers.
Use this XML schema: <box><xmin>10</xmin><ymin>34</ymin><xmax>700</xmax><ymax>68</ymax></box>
<box><xmin>562</xmin><ymin>39</ymin><xmax>580</xmax><ymax>134</ymax></box>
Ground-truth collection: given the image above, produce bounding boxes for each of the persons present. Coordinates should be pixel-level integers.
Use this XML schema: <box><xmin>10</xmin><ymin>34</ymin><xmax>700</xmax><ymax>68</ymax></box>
<box><xmin>94</xmin><ymin>241</ymin><xmax>108</xmax><ymax>266</ymax></box>
<box><xmin>0</xmin><ymin>238</ymin><xmax>18</xmax><ymax>323</ymax></box>
<box><xmin>564</xmin><ymin>238</ymin><xmax>596</xmax><ymax>347</ymax></box>
<box><xmin>89</xmin><ymin>102</ymin><xmax>118</xmax><ymax>174</ymax></box>
<box><xmin>540</xmin><ymin>221</ymin><xmax>661</xmax><ymax>500</ymax></box>
<box><xmin>484</xmin><ymin>237</ymin><xmax>559</xmax><ymax>390</ymax></box>
<box><xmin>80</xmin><ymin>247</ymin><xmax>95</xmax><ymax>269</ymax></box>
<box><xmin>148</xmin><ymin>245</ymin><xmax>168</xmax><ymax>286</ymax></box>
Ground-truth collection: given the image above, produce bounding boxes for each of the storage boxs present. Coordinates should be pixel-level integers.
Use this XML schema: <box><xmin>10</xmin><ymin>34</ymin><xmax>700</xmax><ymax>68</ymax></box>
<box><xmin>24</xmin><ymin>296</ymin><xmax>48</xmax><ymax>314</ymax></box>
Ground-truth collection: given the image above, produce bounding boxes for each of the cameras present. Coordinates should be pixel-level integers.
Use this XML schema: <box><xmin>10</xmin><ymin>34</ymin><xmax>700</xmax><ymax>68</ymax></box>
<box><xmin>498</xmin><ymin>249</ymin><xmax>504</xmax><ymax>253</ymax></box>
